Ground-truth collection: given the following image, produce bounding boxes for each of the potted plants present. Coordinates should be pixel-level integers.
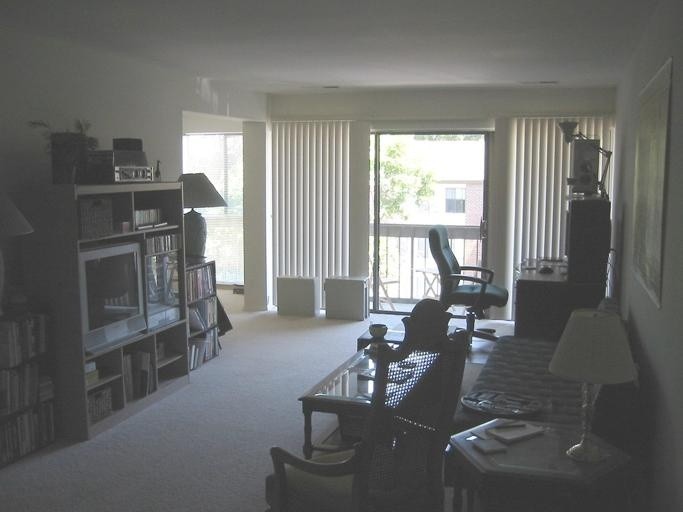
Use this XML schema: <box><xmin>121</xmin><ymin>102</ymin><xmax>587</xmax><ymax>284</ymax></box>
<box><xmin>28</xmin><ymin>116</ymin><xmax>99</xmax><ymax>184</ymax></box>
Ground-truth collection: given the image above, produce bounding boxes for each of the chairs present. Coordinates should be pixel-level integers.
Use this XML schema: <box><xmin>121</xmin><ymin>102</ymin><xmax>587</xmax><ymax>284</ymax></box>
<box><xmin>368</xmin><ymin>254</ymin><xmax>401</xmax><ymax>313</ymax></box>
<box><xmin>428</xmin><ymin>223</ymin><xmax>509</xmax><ymax>356</ymax></box>
<box><xmin>264</xmin><ymin>297</ymin><xmax>469</xmax><ymax>511</ymax></box>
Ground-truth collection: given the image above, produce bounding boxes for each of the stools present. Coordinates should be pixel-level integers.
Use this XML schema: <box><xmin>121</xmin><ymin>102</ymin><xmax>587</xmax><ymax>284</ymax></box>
<box><xmin>357</xmin><ymin>328</ymin><xmax>404</xmax><ymax>349</ymax></box>
<box><xmin>324</xmin><ymin>276</ymin><xmax>370</xmax><ymax>320</ymax></box>
<box><xmin>275</xmin><ymin>275</ymin><xmax>319</xmax><ymax>318</ymax></box>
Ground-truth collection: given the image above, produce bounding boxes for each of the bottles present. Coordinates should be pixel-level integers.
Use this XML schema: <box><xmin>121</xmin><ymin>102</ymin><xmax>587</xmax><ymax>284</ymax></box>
<box><xmin>153</xmin><ymin>160</ymin><xmax>161</xmax><ymax>183</ymax></box>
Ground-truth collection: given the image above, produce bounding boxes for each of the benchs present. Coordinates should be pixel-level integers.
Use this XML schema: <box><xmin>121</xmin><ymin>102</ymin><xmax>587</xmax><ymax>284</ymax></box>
<box><xmin>461</xmin><ymin>297</ymin><xmax>650</xmax><ymax>458</ymax></box>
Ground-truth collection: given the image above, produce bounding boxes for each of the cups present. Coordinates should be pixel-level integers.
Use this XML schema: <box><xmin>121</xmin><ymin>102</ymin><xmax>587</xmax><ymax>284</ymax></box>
<box><xmin>369</xmin><ymin>324</ymin><xmax>387</xmax><ymax>337</ymax></box>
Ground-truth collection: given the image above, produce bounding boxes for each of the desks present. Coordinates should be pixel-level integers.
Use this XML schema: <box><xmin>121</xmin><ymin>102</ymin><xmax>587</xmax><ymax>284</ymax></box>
<box><xmin>442</xmin><ymin>418</ymin><xmax>635</xmax><ymax>511</ymax></box>
<box><xmin>513</xmin><ymin>257</ymin><xmax>610</xmax><ymax>337</ymax></box>
<box><xmin>415</xmin><ymin>267</ymin><xmax>442</xmax><ymax>301</ymax></box>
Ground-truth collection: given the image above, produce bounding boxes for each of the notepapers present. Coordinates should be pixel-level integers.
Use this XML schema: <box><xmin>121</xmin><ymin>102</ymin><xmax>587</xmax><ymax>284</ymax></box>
<box><xmin>471</xmin><ymin>440</ymin><xmax>507</xmax><ymax>455</ymax></box>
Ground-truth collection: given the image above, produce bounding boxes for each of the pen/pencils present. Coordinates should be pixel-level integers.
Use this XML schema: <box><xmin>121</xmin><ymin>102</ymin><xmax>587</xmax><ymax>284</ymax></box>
<box><xmin>496</xmin><ymin>424</ymin><xmax>526</xmax><ymax>428</ymax></box>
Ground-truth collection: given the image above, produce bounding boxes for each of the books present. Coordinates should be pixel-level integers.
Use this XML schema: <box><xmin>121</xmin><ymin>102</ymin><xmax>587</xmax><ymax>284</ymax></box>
<box><xmin>486</xmin><ymin>420</ymin><xmax>545</xmax><ymax>446</ymax></box>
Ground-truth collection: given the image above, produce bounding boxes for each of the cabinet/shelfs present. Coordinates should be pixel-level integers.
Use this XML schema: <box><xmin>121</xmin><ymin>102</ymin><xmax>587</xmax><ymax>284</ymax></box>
<box><xmin>186</xmin><ymin>258</ymin><xmax>220</xmax><ymax>371</ymax></box>
<box><xmin>19</xmin><ymin>179</ymin><xmax>189</xmax><ymax>443</ymax></box>
<box><xmin>0</xmin><ymin>303</ymin><xmax>61</xmax><ymax>469</ymax></box>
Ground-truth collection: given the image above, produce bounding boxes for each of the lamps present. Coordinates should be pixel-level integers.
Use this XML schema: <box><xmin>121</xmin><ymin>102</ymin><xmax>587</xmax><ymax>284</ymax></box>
<box><xmin>558</xmin><ymin>119</ymin><xmax>615</xmax><ymax>198</ymax></box>
<box><xmin>175</xmin><ymin>173</ymin><xmax>228</xmax><ymax>262</ymax></box>
<box><xmin>548</xmin><ymin>307</ymin><xmax>640</xmax><ymax>465</ymax></box>
<box><xmin>0</xmin><ymin>192</ymin><xmax>36</xmax><ymax>306</ymax></box>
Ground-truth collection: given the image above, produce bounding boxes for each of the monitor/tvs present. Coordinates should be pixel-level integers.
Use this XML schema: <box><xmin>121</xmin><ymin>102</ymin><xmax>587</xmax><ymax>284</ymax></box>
<box><xmin>559</xmin><ymin>211</ymin><xmax>568</xmax><ymax>274</ymax></box>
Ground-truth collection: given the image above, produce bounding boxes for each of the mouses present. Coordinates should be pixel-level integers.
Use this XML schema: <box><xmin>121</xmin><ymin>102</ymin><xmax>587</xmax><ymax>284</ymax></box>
<box><xmin>539</xmin><ymin>266</ymin><xmax>553</xmax><ymax>273</ymax></box>
